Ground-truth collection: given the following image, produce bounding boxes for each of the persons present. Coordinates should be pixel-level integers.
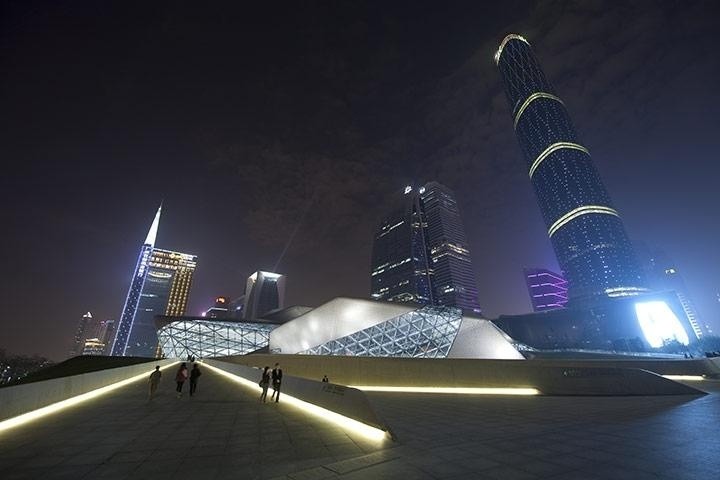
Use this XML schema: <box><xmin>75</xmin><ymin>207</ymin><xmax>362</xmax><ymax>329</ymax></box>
<box><xmin>260</xmin><ymin>367</ymin><xmax>270</xmax><ymax>403</ymax></box>
<box><xmin>148</xmin><ymin>366</ymin><xmax>161</xmax><ymax>400</ymax></box>
<box><xmin>175</xmin><ymin>365</ymin><xmax>187</xmax><ymax>399</ymax></box>
<box><xmin>189</xmin><ymin>364</ymin><xmax>202</xmax><ymax>399</ymax></box>
<box><xmin>271</xmin><ymin>363</ymin><xmax>282</xmax><ymax>402</ymax></box>
<box><xmin>322</xmin><ymin>375</ymin><xmax>328</xmax><ymax>382</ymax></box>
